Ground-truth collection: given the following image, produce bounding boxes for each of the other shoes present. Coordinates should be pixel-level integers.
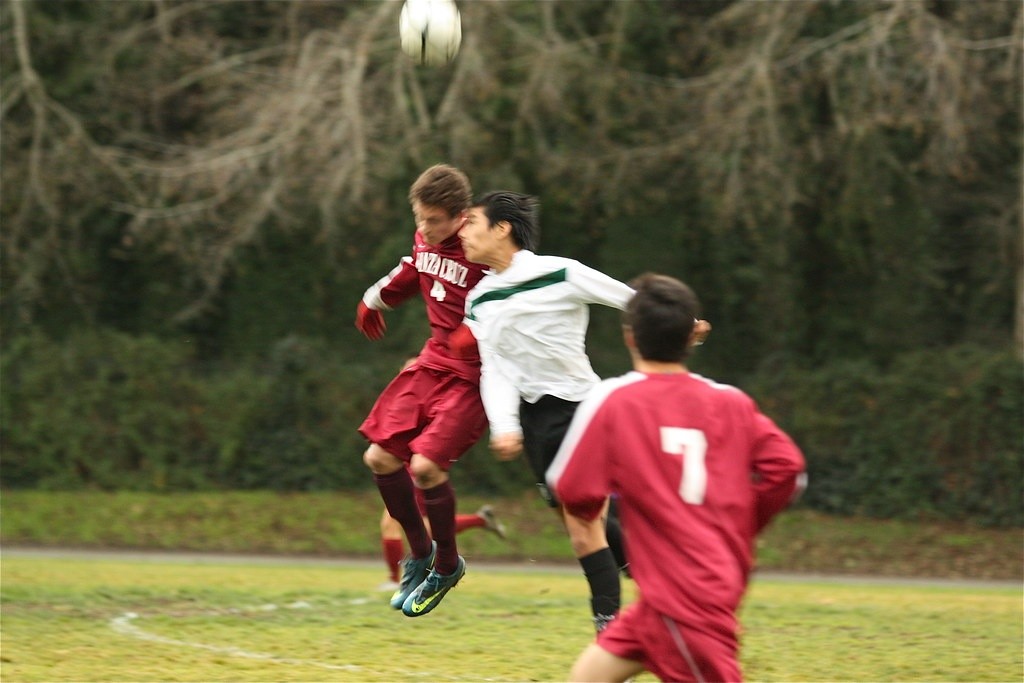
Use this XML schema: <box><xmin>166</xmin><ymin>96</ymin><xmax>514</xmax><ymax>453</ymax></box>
<box><xmin>594</xmin><ymin>613</ymin><xmax>615</xmax><ymax>635</ymax></box>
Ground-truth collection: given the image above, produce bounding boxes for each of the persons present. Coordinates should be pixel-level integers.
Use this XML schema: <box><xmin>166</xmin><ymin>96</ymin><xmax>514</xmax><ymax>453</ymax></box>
<box><xmin>353</xmin><ymin>163</ymin><xmax>714</xmax><ymax>631</ymax></box>
<box><xmin>543</xmin><ymin>268</ymin><xmax>808</xmax><ymax>682</ymax></box>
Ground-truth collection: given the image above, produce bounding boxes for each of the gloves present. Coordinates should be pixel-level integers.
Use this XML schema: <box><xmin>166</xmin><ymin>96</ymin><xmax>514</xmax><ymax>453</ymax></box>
<box><xmin>356</xmin><ymin>300</ymin><xmax>386</xmax><ymax>340</ymax></box>
<box><xmin>448</xmin><ymin>324</ymin><xmax>478</xmax><ymax>357</ymax></box>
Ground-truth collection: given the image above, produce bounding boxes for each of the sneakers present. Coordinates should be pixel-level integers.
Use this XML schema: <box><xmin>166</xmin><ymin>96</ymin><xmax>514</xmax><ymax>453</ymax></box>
<box><xmin>477</xmin><ymin>506</ymin><xmax>507</xmax><ymax>539</ymax></box>
<box><xmin>390</xmin><ymin>541</ymin><xmax>436</xmax><ymax>611</ymax></box>
<box><xmin>402</xmin><ymin>555</ymin><xmax>466</xmax><ymax>618</ymax></box>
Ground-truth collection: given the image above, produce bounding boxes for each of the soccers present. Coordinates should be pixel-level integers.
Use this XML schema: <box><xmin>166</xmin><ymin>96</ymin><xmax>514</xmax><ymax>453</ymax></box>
<box><xmin>399</xmin><ymin>0</ymin><xmax>462</xmax><ymax>69</ymax></box>
